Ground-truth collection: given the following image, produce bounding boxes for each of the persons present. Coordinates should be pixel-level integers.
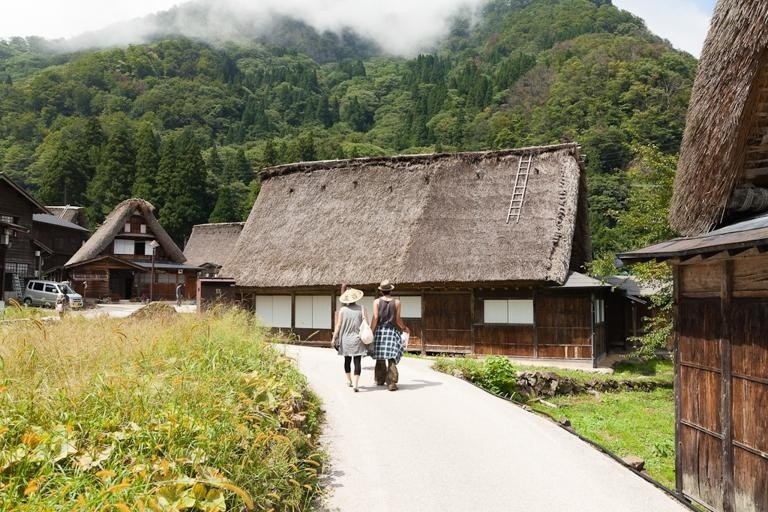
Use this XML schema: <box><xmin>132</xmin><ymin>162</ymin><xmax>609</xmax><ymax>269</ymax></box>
<box><xmin>176</xmin><ymin>283</ymin><xmax>183</xmax><ymax>306</ymax></box>
<box><xmin>331</xmin><ymin>287</ymin><xmax>369</xmax><ymax>391</ymax></box>
<box><xmin>56</xmin><ymin>288</ymin><xmax>70</xmax><ymax>318</ymax></box>
<box><xmin>369</xmin><ymin>279</ymin><xmax>408</xmax><ymax>391</ymax></box>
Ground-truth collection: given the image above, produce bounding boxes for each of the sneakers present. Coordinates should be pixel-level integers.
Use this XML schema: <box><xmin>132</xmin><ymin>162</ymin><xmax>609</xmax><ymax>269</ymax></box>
<box><xmin>347</xmin><ymin>378</ymin><xmax>399</xmax><ymax>392</ymax></box>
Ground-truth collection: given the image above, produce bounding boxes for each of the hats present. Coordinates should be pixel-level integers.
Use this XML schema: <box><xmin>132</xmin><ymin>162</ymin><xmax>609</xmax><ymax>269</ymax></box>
<box><xmin>339</xmin><ymin>287</ymin><xmax>364</xmax><ymax>303</ymax></box>
<box><xmin>378</xmin><ymin>279</ymin><xmax>394</xmax><ymax>291</ymax></box>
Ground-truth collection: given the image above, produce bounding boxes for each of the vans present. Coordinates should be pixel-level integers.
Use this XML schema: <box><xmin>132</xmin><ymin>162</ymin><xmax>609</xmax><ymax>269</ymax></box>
<box><xmin>22</xmin><ymin>280</ymin><xmax>83</xmax><ymax>311</ymax></box>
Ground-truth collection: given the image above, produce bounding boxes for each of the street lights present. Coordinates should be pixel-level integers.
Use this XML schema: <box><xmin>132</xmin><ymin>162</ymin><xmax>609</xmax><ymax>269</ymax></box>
<box><xmin>149</xmin><ymin>240</ymin><xmax>160</xmax><ymax>302</ymax></box>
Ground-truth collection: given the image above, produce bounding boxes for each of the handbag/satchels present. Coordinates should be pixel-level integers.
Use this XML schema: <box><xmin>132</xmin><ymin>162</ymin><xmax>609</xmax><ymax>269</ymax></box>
<box><xmin>359</xmin><ymin>305</ymin><xmax>374</xmax><ymax>345</ymax></box>
